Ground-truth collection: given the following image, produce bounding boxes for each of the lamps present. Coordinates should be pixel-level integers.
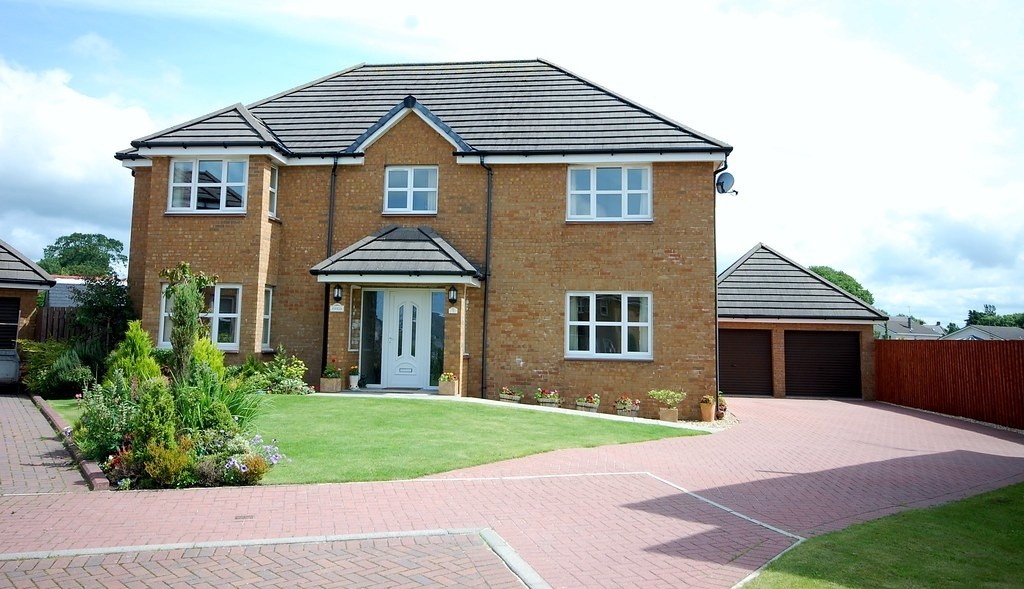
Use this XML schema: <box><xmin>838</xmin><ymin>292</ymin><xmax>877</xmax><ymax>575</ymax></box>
<box><xmin>448</xmin><ymin>285</ymin><xmax>457</xmax><ymax>305</ymax></box>
<box><xmin>333</xmin><ymin>283</ymin><xmax>342</xmax><ymax>303</ymax></box>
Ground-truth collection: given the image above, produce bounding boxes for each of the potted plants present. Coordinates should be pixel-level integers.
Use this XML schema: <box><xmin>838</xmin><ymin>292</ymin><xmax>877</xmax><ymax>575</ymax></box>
<box><xmin>648</xmin><ymin>389</ymin><xmax>686</xmax><ymax>422</ymax></box>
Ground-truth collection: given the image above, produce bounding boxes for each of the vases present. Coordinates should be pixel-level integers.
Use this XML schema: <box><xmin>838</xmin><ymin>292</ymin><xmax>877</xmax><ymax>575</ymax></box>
<box><xmin>320</xmin><ymin>378</ymin><xmax>341</xmax><ymax>393</ymax></box>
<box><xmin>349</xmin><ymin>375</ymin><xmax>359</xmax><ymax>391</ymax></box>
<box><xmin>499</xmin><ymin>394</ymin><xmax>521</xmax><ymax>403</ymax></box>
<box><xmin>439</xmin><ymin>381</ymin><xmax>458</xmax><ymax>395</ymax></box>
<box><xmin>717</xmin><ymin>414</ymin><xmax>724</xmax><ymax>419</ymax></box>
<box><xmin>617</xmin><ymin>406</ymin><xmax>639</xmax><ymax>416</ymax></box>
<box><xmin>539</xmin><ymin>399</ymin><xmax>560</xmax><ymax>407</ymax></box>
<box><xmin>576</xmin><ymin>402</ymin><xmax>599</xmax><ymax>412</ymax></box>
<box><xmin>700</xmin><ymin>403</ymin><xmax>715</xmax><ymax>422</ymax></box>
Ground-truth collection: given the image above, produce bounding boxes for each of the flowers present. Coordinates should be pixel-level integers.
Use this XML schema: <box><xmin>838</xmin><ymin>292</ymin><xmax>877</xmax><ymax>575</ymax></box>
<box><xmin>716</xmin><ymin>401</ymin><xmax>725</xmax><ymax>416</ymax></box>
<box><xmin>700</xmin><ymin>395</ymin><xmax>714</xmax><ymax>404</ymax></box>
<box><xmin>577</xmin><ymin>393</ymin><xmax>600</xmax><ymax>404</ymax></box>
<box><xmin>322</xmin><ymin>359</ymin><xmax>342</xmax><ymax>378</ymax></box>
<box><xmin>536</xmin><ymin>388</ymin><xmax>559</xmax><ymax>399</ymax></box>
<box><xmin>500</xmin><ymin>386</ymin><xmax>524</xmax><ymax>398</ymax></box>
<box><xmin>349</xmin><ymin>365</ymin><xmax>358</xmax><ymax>375</ymax></box>
<box><xmin>612</xmin><ymin>396</ymin><xmax>641</xmax><ymax>412</ymax></box>
<box><xmin>438</xmin><ymin>372</ymin><xmax>458</xmax><ymax>382</ymax></box>
